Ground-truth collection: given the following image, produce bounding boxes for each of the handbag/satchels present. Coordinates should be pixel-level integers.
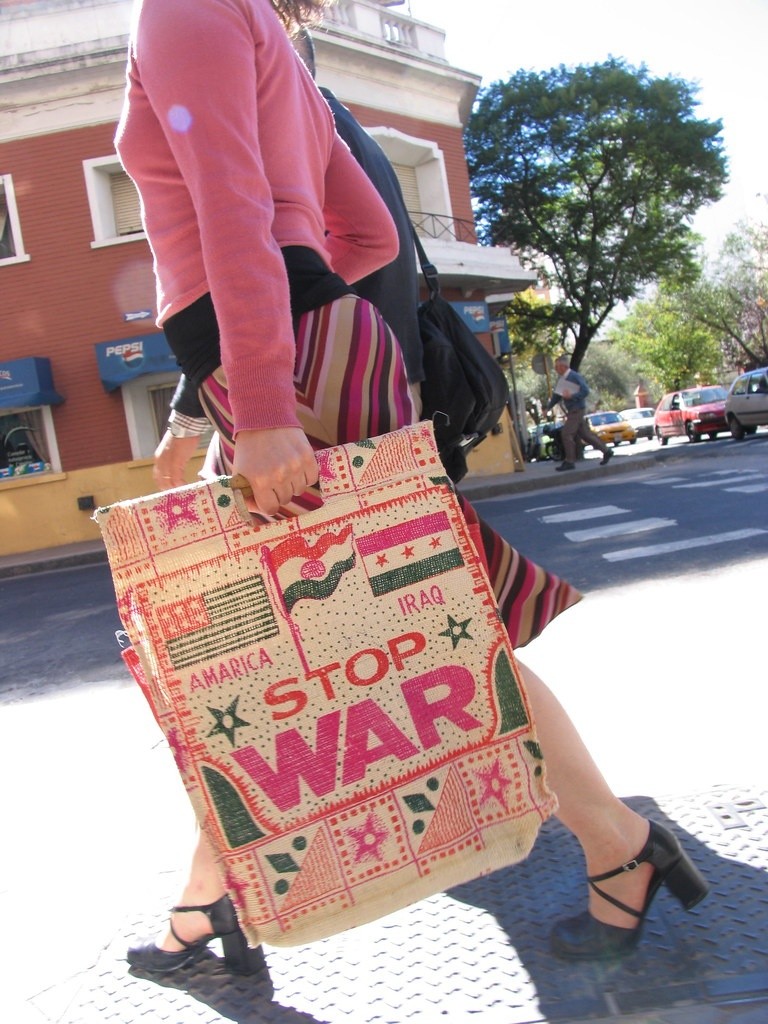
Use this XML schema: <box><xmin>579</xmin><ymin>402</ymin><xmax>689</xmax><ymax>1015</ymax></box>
<box><xmin>89</xmin><ymin>420</ymin><xmax>554</xmax><ymax>946</ymax></box>
<box><xmin>417</xmin><ymin>298</ymin><xmax>508</xmax><ymax>465</ymax></box>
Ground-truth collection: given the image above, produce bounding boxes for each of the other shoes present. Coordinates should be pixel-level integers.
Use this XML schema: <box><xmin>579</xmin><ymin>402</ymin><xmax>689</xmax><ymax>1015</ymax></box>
<box><xmin>555</xmin><ymin>462</ymin><xmax>575</xmax><ymax>470</ymax></box>
<box><xmin>600</xmin><ymin>449</ymin><xmax>613</xmax><ymax>465</ymax></box>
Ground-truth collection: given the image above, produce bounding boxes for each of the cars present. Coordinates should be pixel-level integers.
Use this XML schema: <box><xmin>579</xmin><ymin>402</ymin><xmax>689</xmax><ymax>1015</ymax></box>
<box><xmin>651</xmin><ymin>384</ymin><xmax>729</xmax><ymax>445</ymax></box>
<box><xmin>724</xmin><ymin>366</ymin><xmax>768</xmax><ymax>441</ymax></box>
<box><xmin>581</xmin><ymin>411</ymin><xmax>637</xmax><ymax>448</ymax></box>
<box><xmin>528</xmin><ymin>420</ymin><xmax>569</xmax><ymax>459</ymax></box>
<box><xmin>618</xmin><ymin>407</ymin><xmax>656</xmax><ymax>440</ymax></box>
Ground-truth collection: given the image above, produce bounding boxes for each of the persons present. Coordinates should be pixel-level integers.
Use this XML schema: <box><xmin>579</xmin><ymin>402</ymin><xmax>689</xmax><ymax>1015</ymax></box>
<box><xmin>125</xmin><ymin>0</ymin><xmax>710</xmax><ymax>975</ymax></box>
<box><xmin>543</xmin><ymin>354</ymin><xmax>614</xmax><ymax>471</ymax></box>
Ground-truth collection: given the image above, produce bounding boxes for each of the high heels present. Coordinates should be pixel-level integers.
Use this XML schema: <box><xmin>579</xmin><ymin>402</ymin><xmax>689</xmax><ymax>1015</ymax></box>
<box><xmin>127</xmin><ymin>893</ymin><xmax>265</xmax><ymax>973</ymax></box>
<box><xmin>550</xmin><ymin>819</ymin><xmax>708</xmax><ymax>961</ymax></box>
